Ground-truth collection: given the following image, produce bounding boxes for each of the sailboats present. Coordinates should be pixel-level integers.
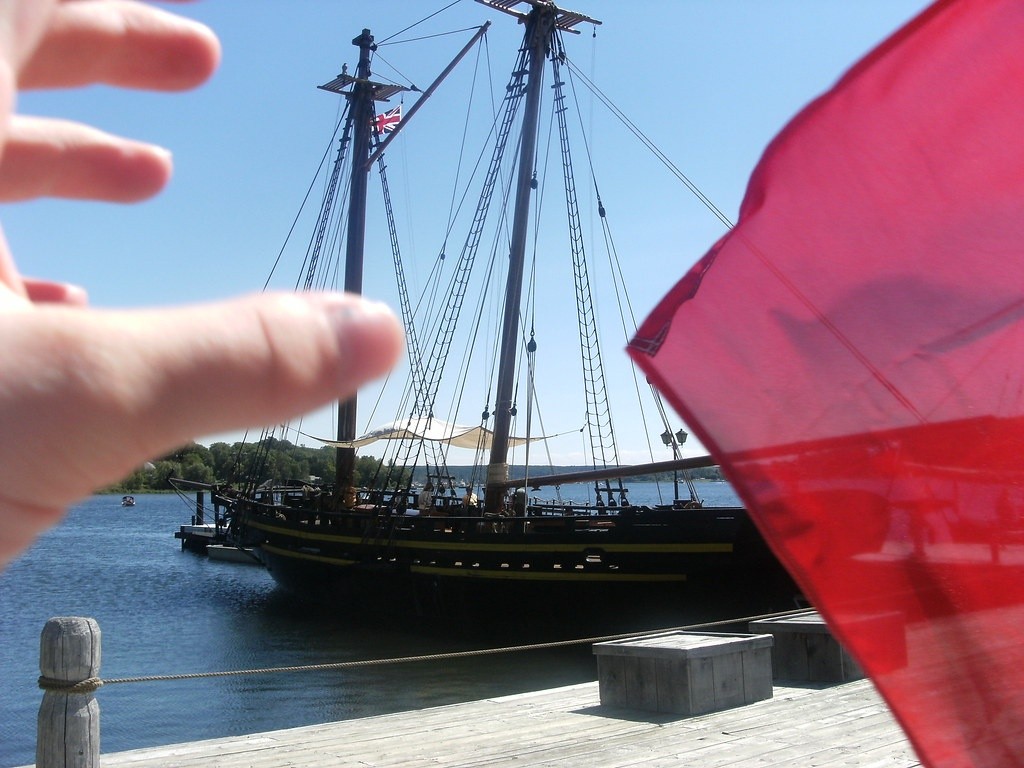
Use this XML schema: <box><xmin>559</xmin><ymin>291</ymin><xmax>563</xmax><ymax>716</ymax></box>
<box><xmin>155</xmin><ymin>0</ymin><xmax>891</xmax><ymax>611</ymax></box>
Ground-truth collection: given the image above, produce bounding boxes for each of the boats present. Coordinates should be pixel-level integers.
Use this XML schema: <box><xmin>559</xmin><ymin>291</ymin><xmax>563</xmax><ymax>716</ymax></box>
<box><xmin>121</xmin><ymin>495</ymin><xmax>136</xmax><ymax>507</ymax></box>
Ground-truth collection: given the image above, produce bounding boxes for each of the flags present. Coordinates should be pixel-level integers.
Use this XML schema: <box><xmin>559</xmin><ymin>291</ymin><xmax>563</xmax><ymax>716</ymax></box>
<box><xmin>370</xmin><ymin>106</ymin><xmax>402</xmax><ymax>134</ymax></box>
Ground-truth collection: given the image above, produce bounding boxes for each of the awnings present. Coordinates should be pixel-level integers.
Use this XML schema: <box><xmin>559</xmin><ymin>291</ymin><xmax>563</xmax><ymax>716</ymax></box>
<box><xmin>279</xmin><ymin>411</ymin><xmax>583</xmax><ymax>449</ymax></box>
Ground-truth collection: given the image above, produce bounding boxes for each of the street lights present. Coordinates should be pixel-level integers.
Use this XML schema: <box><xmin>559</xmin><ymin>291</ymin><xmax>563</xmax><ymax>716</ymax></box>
<box><xmin>659</xmin><ymin>427</ymin><xmax>689</xmax><ymax>500</ymax></box>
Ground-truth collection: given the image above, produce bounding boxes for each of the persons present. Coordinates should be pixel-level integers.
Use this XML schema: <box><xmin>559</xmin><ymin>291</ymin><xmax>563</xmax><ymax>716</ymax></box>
<box><xmin>507</xmin><ymin>496</ymin><xmax>513</xmax><ymax>510</ymax></box>
<box><xmin>419</xmin><ymin>481</ymin><xmax>434</xmax><ymax>508</ymax></box>
<box><xmin>463</xmin><ymin>484</ymin><xmax>477</xmax><ymax>508</ymax></box>
<box><xmin>0</xmin><ymin>0</ymin><xmax>408</xmax><ymax>573</ymax></box>
